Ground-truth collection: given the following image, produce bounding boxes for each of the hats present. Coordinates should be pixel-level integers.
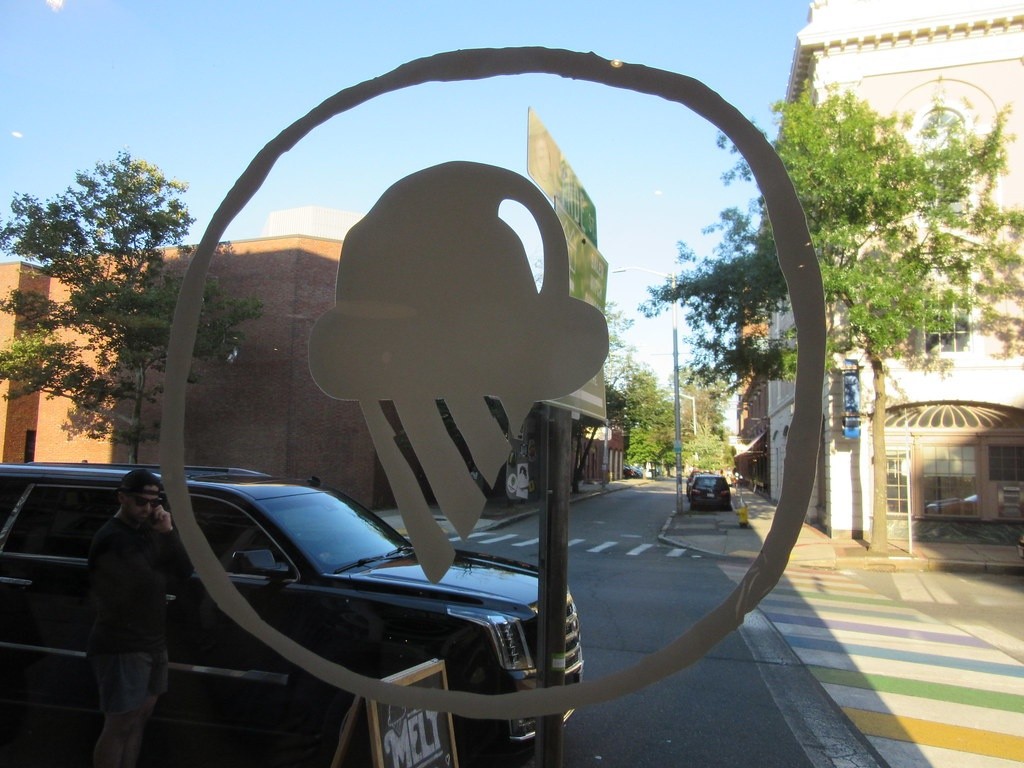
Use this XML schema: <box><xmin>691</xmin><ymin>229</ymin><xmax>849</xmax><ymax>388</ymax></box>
<box><xmin>110</xmin><ymin>470</ymin><xmax>161</xmax><ymax>495</ymax></box>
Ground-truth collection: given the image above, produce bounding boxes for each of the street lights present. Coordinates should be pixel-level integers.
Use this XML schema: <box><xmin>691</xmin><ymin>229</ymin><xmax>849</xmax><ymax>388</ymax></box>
<box><xmin>613</xmin><ymin>267</ymin><xmax>684</xmax><ymax>514</ymax></box>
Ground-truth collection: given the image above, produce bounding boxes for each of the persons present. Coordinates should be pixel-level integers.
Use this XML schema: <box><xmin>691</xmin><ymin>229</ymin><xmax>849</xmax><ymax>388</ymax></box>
<box><xmin>88</xmin><ymin>469</ymin><xmax>194</xmax><ymax>768</ymax></box>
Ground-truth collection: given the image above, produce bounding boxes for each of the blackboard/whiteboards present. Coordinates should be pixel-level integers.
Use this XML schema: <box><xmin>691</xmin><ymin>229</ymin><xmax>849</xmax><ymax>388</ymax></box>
<box><xmin>377</xmin><ymin>671</ymin><xmax>456</xmax><ymax>768</ymax></box>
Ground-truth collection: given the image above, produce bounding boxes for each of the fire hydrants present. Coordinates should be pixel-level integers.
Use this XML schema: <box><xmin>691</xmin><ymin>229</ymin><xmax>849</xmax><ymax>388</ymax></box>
<box><xmin>735</xmin><ymin>497</ymin><xmax>750</xmax><ymax>530</ymax></box>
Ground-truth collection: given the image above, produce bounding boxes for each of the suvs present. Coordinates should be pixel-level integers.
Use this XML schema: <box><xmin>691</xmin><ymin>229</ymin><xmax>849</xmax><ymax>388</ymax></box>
<box><xmin>0</xmin><ymin>462</ymin><xmax>588</xmax><ymax>768</ymax></box>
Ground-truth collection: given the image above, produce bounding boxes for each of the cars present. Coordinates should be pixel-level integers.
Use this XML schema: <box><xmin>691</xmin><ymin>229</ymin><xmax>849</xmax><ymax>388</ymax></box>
<box><xmin>623</xmin><ymin>464</ymin><xmax>644</xmax><ymax>480</ymax></box>
<box><xmin>925</xmin><ymin>494</ymin><xmax>1005</xmax><ymax>517</ymax></box>
<box><xmin>687</xmin><ymin>473</ymin><xmax>733</xmax><ymax>511</ymax></box>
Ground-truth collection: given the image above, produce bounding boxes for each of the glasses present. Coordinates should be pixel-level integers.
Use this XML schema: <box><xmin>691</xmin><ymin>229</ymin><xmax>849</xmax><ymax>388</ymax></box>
<box><xmin>131</xmin><ymin>493</ymin><xmax>164</xmax><ymax>508</ymax></box>
